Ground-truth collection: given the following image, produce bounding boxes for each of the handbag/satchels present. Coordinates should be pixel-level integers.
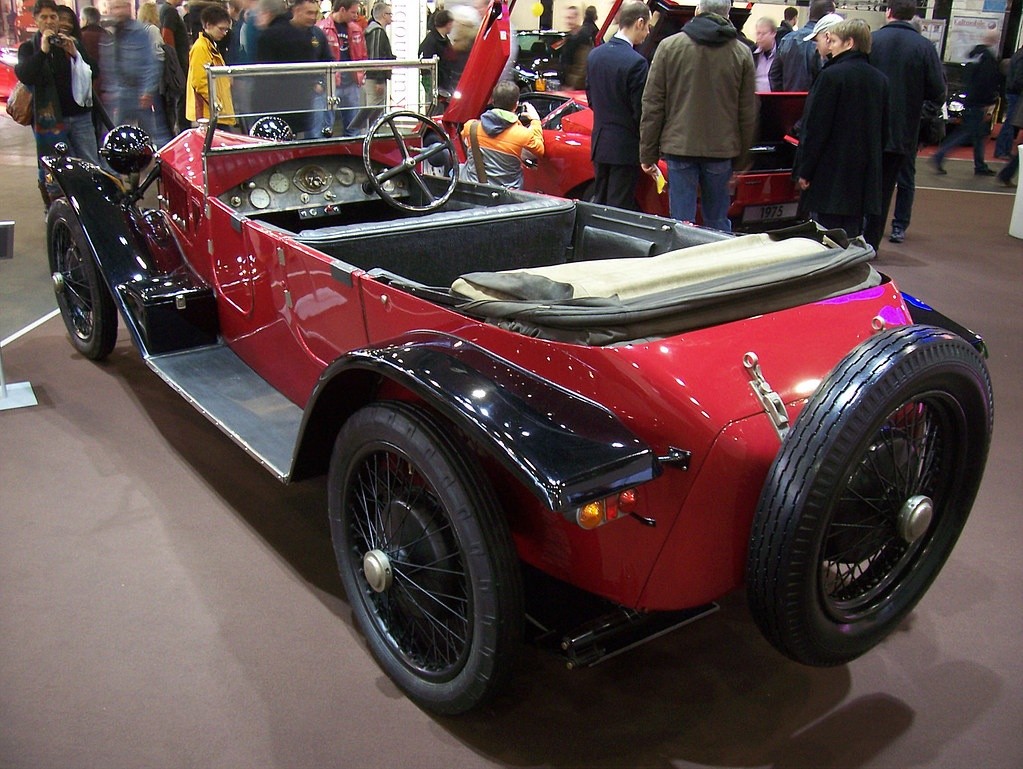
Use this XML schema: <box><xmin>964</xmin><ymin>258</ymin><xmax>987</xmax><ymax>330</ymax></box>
<box><xmin>6</xmin><ymin>80</ymin><xmax>35</xmax><ymax>126</ymax></box>
<box><xmin>69</xmin><ymin>49</ymin><xmax>93</xmax><ymax>107</ymax></box>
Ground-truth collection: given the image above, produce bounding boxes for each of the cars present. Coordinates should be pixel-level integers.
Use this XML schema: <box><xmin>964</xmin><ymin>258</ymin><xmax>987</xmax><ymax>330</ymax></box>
<box><xmin>507</xmin><ymin>30</ymin><xmax>594</xmax><ymax>95</ymax></box>
<box><xmin>936</xmin><ymin>62</ymin><xmax>1001</xmax><ymax>141</ymax></box>
<box><xmin>44</xmin><ymin>55</ymin><xmax>993</xmax><ymax>718</ymax></box>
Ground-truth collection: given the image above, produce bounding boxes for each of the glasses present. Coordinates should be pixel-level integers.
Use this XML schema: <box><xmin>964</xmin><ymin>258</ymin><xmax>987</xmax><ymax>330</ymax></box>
<box><xmin>215</xmin><ymin>22</ymin><xmax>230</xmax><ymax>33</ymax></box>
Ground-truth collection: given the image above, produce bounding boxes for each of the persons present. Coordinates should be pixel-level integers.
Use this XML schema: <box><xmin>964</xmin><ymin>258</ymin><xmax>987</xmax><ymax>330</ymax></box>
<box><xmin>13</xmin><ymin>1</ymin><xmax>1023</xmax><ymax>260</ymax></box>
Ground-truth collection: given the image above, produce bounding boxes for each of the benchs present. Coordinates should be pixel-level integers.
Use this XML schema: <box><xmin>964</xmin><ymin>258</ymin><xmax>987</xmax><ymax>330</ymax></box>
<box><xmin>254</xmin><ymin>201</ymin><xmax>828</xmax><ymax>308</ymax></box>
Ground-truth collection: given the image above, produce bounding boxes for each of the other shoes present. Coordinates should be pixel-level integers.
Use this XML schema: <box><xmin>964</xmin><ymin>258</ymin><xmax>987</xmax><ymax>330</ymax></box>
<box><xmin>999</xmin><ymin>174</ymin><xmax>1017</xmax><ymax>187</ymax></box>
<box><xmin>976</xmin><ymin>170</ymin><xmax>997</xmax><ymax>176</ymax></box>
<box><xmin>997</xmin><ymin>152</ymin><xmax>1013</xmax><ymax>159</ymax></box>
<box><xmin>932</xmin><ymin>154</ymin><xmax>947</xmax><ymax>174</ymax></box>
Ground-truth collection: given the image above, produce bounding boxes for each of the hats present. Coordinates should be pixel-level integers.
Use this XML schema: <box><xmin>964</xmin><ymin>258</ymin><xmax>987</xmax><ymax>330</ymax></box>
<box><xmin>803</xmin><ymin>13</ymin><xmax>844</xmax><ymax>41</ymax></box>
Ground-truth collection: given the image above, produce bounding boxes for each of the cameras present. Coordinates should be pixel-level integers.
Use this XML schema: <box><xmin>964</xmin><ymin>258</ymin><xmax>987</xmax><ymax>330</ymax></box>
<box><xmin>515</xmin><ymin>105</ymin><xmax>525</xmax><ymax>114</ymax></box>
<box><xmin>49</xmin><ymin>34</ymin><xmax>65</xmax><ymax>45</ymax></box>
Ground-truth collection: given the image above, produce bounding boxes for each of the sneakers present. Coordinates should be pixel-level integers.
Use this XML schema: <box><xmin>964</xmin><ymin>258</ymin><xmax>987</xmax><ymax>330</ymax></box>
<box><xmin>889</xmin><ymin>227</ymin><xmax>904</xmax><ymax>242</ymax></box>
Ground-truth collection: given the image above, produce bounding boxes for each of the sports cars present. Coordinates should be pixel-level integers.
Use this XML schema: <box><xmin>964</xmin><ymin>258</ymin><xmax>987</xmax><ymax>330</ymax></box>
<box><xmin>411</xmin><ymin>89</ymin><xmax>809</xmax><ymax>233</ymax></box>
